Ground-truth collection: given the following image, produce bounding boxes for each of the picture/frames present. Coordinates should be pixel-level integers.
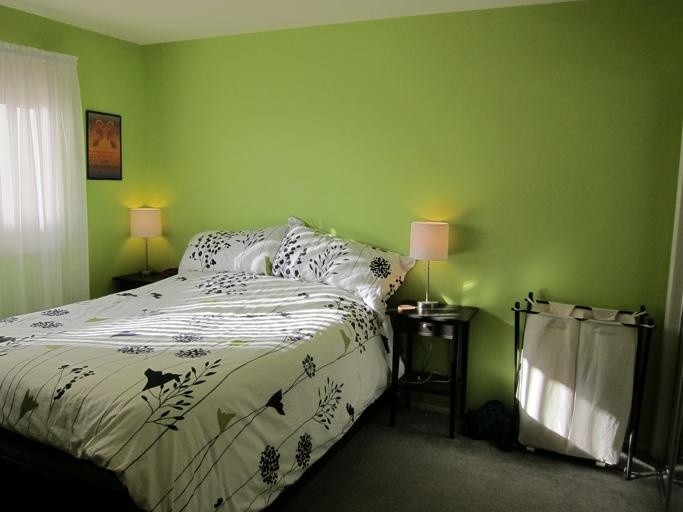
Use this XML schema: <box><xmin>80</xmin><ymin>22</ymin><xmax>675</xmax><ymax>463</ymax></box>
<box><xmin>85</xmin><ymin>110</ymin><xmax>122</xmax><ymax>180</ymax></box>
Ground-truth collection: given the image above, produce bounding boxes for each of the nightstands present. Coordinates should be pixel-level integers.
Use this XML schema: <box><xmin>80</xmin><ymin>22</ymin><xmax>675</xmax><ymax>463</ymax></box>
<box><xmin>113</xmin><ymin>274</ymin><xmax>169</xmax><ymax>291</ymax></box>
<box><xmin>385</xmin><ymin>299</ymin><xmax>479</xmax><ymax>441</ymax></box>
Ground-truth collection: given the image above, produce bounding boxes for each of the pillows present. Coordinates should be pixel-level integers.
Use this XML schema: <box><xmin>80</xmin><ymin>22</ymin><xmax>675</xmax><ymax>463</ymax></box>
<box><xmin>273</xmin><ymin>216</ymin><xmax>415</xmax><ymax>322</ymax></box>
<box><xmin>177</xmin><ymin>224</ymin><xmax>291</xmax><ymax>275</ymax></box>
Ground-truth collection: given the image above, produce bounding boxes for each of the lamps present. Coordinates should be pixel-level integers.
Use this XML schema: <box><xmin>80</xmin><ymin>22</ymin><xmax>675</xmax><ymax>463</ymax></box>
<box><xmin>411</xmin><ymin>221</ymin><xmax>449</xmax><ymax>308</ymax></box>
<box><xmin>130</xmin><ymin>207</ymin><xmax>162</xmax><ymax>275</ymax></box>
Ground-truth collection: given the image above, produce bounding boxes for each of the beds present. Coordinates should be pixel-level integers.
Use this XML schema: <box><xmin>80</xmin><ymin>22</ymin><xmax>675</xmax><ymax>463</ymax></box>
<box><xmin>0</xmin><ymin>216</ymin><xmax>417</xmax><ymax>512</ymax></box>
